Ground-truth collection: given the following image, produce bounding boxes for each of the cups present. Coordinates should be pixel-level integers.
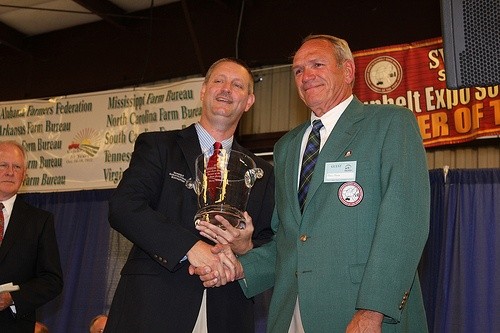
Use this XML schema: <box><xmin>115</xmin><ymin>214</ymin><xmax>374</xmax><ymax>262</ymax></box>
<box><xmin>186</xmin><ymin>149</ymin><xmax>263</xmax><ymax>230</ymax></box>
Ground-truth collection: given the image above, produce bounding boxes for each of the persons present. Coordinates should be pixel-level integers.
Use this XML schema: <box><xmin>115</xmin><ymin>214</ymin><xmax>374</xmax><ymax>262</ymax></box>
<box><xmin>0</xmin><ymin>139</ymin><xmax>63</xmax><ymax>333</ymax></box>
<box><xmin>35</xmin><ymin>321</ymin><xmax>48</xmax><ymax>333</ymax></box>
<box><xmin>90</xmin><ymin>315</ymin><xmax>108</xmax><ymax>333</ymax></box>
<box><xmin>103</xmin><ymin>58</ymin><xmax>275</xmax><ymax>333</ymax></box>
<box><xmin>189</xmin><ymin>35</ymin><xmax>431</xmax><ymax>333</ymax></box>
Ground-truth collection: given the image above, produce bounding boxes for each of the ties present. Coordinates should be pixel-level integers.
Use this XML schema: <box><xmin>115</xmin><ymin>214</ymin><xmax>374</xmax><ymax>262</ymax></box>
<box><xmin>0</xmin><ymin>203</ymin><xmax>5</xmax><ymax>243</ymax></box>
<box><xmin>205</xmin><ymin>142</ymin><xmax>223</xmax><ymax>205</ymax></box>
<box><xmin>298</xmin><ymin>119</ymin><xmax>324</xmax><ymax>215</ymax></box>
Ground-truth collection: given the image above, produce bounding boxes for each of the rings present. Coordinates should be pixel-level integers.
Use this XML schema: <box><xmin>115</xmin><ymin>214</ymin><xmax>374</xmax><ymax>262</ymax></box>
<box><xmin>213</xmin><ymin>234</ymin><xmax>218</xmax><ymax>241</ymax></box>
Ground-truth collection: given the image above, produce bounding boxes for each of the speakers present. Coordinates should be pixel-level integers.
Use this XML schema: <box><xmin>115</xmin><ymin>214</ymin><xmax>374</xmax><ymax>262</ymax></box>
<box><xmin>440</xmin><ymin>0</ymin><xmax>500</xmax><ymax>90</ymax></box>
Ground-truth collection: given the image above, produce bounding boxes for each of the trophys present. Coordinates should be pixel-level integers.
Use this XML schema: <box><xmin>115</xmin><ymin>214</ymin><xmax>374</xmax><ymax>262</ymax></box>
<box><xmin>185</xmin><ymin>148</ymin><xmax>263</xmax><ymax>231</ymax></box>
